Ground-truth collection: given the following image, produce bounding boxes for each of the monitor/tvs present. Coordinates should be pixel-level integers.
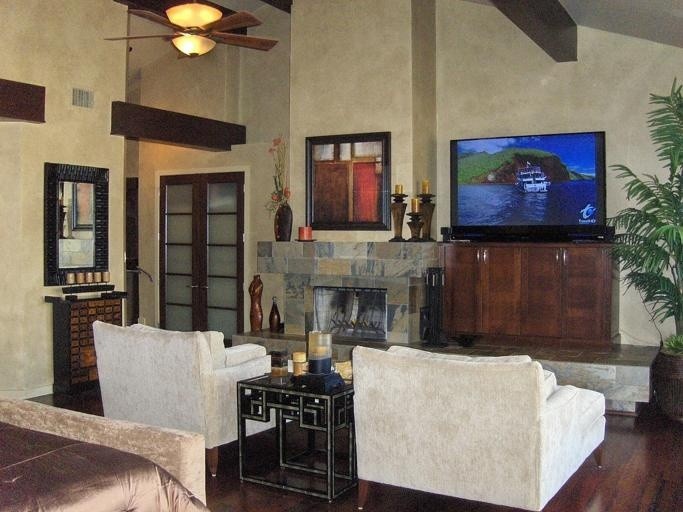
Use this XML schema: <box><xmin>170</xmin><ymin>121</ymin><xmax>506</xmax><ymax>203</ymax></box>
<box><xmin>450</xmin><ymin>131</ymin><xmax>606</xmax><ymax>242</ymax></box>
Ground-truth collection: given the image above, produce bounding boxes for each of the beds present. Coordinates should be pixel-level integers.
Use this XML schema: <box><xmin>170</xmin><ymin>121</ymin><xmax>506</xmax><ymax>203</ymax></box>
<box><xmin>0</xmin><ymin>396</ymin><xmax>209</xmax><ymax>512</ymax></box>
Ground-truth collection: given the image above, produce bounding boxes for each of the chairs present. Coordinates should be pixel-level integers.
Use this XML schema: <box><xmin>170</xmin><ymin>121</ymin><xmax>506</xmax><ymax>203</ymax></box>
<box><xmin>352</xmin><ymin>345</ymin><xmax>606</xmax><ymax>512</ymax></box>
<box><xmin>93</xmin><ymin>320</ymin><xmax>276</xmax><ymax>479</ymax></box>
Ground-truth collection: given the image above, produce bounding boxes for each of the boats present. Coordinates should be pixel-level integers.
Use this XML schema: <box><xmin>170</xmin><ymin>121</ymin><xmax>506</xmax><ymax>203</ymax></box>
<box><xmin>515</xmin><ymin>160</ymin><xmax>551</xmax><ymax>194</ymax></box>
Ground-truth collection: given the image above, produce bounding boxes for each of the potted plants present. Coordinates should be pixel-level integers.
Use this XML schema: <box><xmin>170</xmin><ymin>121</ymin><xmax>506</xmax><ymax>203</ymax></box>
<box><xmin>604</xmin><ymin>78</ymin><xmax>683</xmax><ymax>424</ymax></box>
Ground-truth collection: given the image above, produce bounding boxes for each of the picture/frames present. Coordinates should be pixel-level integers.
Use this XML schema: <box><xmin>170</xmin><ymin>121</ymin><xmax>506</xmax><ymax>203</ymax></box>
<box><xmin>305</xmin><ymin>131</ymin><xmax>391</xmax><ymax>231</ymax></box>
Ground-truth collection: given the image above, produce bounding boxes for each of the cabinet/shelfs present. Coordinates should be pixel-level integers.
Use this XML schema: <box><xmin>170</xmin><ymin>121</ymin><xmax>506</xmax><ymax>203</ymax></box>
<box><xmin>438</xmin><ymin>241</ymin><xmax>520</xmax><ymax>352</ymax></box>
<box><xmin>521</xmin><ymin>242</ymin><xmax>621</xmax><ymax>355</ymax></box>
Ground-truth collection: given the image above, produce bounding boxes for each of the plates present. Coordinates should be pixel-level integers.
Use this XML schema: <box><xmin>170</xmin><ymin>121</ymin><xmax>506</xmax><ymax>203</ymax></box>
<box><xmin>294</xmin><ymin>238</ymin><xmax>316</xmax><ymax>242</ymax></box>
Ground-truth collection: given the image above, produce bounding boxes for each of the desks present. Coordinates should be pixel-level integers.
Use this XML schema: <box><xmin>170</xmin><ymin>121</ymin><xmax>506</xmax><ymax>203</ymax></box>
<box><xmin>237</xmin><ymin>372</ymin><xmax>356</xmax><ymax>503</ymax></box>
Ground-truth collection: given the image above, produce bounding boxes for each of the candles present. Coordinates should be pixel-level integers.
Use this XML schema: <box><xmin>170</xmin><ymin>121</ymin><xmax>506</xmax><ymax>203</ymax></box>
<box><xmin>412</xmin><ymin>199</ymin><xmax>420</xmax><ymax>212</ymax></box>
<box><xmin>86</xmin><ymin>272</ymin><xmax>92</xmax><ymax>282</ymax></box>
<box><xmin>395</xmin><ymin>184</ymin><xmax>403</xmax><ymax>194</ymax></box>
<box><xmin>77</xmin><ymin>273</ymin><xmax>84</xmax><ymax>283</ymax></box>
<box><xmin>67</xmin><ymin>273</ymin><xmax>74</xmax><ymax>283</ymax></box>
<box><xmin>94</xmin><ymin>272</ymin><xmax>101</xmax><ymax>282</ymax></box>
<box><xmin>423</xmin><ymin>180</ymin><xmax>429</xmax><ymax>193</ymax></box>
<box><xmin>103</xmin><ymin>272</ymin><xmax>110</xmax><ymax>282</ymax></box>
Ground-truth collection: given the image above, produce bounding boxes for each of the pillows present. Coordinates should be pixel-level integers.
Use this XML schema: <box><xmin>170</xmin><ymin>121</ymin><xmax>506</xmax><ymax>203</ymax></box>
<box><xmin>131</xmin><ymin>323</ymin><xmax>226</xmax><ymax>370</ymax></box>
<box><xmin>388</xmin><ymin>346</ymin><xmax>531</xmax><ymax>364</ymax></box>
<box><xmin>543</xmin><ymin>369</ymin><xmax>556</xmax><ymax>399</ymax></box>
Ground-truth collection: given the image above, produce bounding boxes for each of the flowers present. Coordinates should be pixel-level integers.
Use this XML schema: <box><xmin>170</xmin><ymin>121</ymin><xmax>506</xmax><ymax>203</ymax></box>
<box><xmin>265</xmin><ymin>129</ymin><xmax>290</xmax><ymax>213</ymax></box>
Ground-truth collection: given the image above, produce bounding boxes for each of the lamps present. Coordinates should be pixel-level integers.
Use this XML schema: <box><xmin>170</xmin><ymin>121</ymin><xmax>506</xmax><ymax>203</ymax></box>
<box><xmin>166</xmin><ymin>4</ymin><xmax>223</xmax><ymax>57</ymax></box>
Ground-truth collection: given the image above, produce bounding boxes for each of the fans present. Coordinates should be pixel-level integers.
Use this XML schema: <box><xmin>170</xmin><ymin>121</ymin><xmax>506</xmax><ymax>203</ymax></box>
<box><xmin>104</xmin><ymin>0</ymin><xmax>278</xmax><ymax>59</ymax></box>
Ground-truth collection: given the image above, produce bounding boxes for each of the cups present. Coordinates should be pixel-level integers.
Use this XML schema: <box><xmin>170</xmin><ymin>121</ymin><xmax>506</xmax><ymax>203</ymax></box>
<box><xmin>66</xmin><ymin>271</ymin><xmax>109</xmax><ymax>283</ymax></box>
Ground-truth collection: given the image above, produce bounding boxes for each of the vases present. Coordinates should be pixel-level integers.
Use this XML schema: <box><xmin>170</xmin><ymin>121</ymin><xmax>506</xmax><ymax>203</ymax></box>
<box><xmin>274</xmin><ymin>204</ymin><xmax>292</xmax><ymax>241</ymax></box>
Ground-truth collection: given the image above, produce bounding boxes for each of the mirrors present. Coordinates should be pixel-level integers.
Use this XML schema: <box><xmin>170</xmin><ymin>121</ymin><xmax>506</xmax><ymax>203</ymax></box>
<box><xmin>44</xmin><ymin>162</ymin><xmax>109</xmax><ymax>285</ymax></box>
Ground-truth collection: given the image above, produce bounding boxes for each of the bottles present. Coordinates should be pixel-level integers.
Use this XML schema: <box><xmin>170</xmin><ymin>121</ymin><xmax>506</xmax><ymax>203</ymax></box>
<box><xmin>272</xmin><ymin>330</ymin><xmax>332</xmax><ymax>377</ymax></box>
